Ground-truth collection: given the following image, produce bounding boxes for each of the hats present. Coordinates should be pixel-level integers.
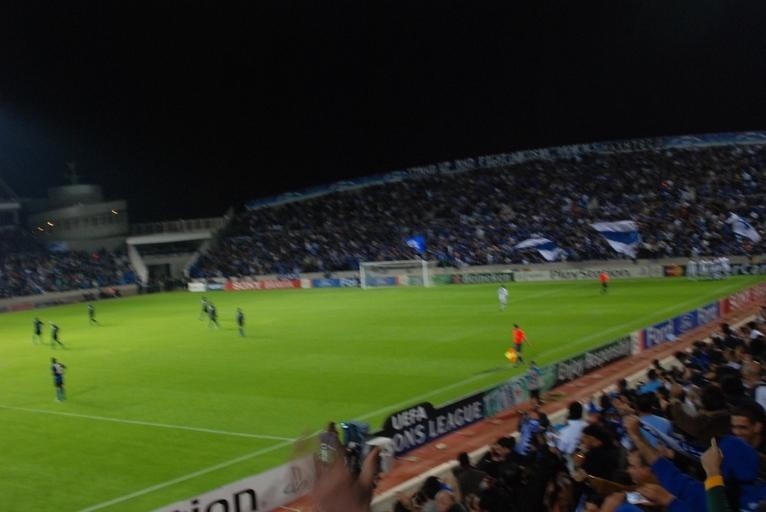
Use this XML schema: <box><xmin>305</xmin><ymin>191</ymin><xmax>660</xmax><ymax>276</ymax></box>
<box><xmin>719</xmin><ymin>436</ymin><xmax>759</xmax><ymax>479</ymax></box>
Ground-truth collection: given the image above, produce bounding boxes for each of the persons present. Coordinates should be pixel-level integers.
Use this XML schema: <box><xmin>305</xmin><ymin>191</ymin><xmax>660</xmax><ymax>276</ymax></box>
<box><xmin>30</xmin><ymin>316</ymin><xmax>42</xmax><ymax>343</ymax></box>
<box><xmin>205</xmin><ymin>303</ymin><xmax>219</xmax><ymax>329</ymax></box>
<box><xmin>47</xmin><ymin>320</ymin><xmax>61</xmax><ymax>349</ymax></box>
<box><xmin>497</xmin><ymin>283</ymin><xmax>508</xmax><ymax>312</ymax></box>
<box><xmin>304</xmin><ymin>310</ymin><xmax>766</xmax><ymax>512</ymax></box>
<box><xmin>235</xmin><ymin>306</ymin><xmax>245</xmax><ymax>338</ymax></box>
<box><xmin>1</xmin><ymin>224</ymin><xmax>142</xmax><ymax>302</ymax></box>
<box><xmin>198</xmin><ymin>296</ymin><xmax>209</xmax><ymax>318</ymax></box>
<box><xmin>86</xmin><ymin>303</ymin><xmax>100</xmax><ymax>327</ymax></box>
<box><xmin>190</xmin><ymin>140</ymin><xmax>766</xmax><ymax>279</ymax></box>
<box><xmin>50</xmin><ymin>356</ymin><xmax>66</xmax><ymax>405</ymax></box>
<box><xmin>598</xmin><ymin>269</ymin><xmax>610</xmax><ymax>294</ymax></box>
